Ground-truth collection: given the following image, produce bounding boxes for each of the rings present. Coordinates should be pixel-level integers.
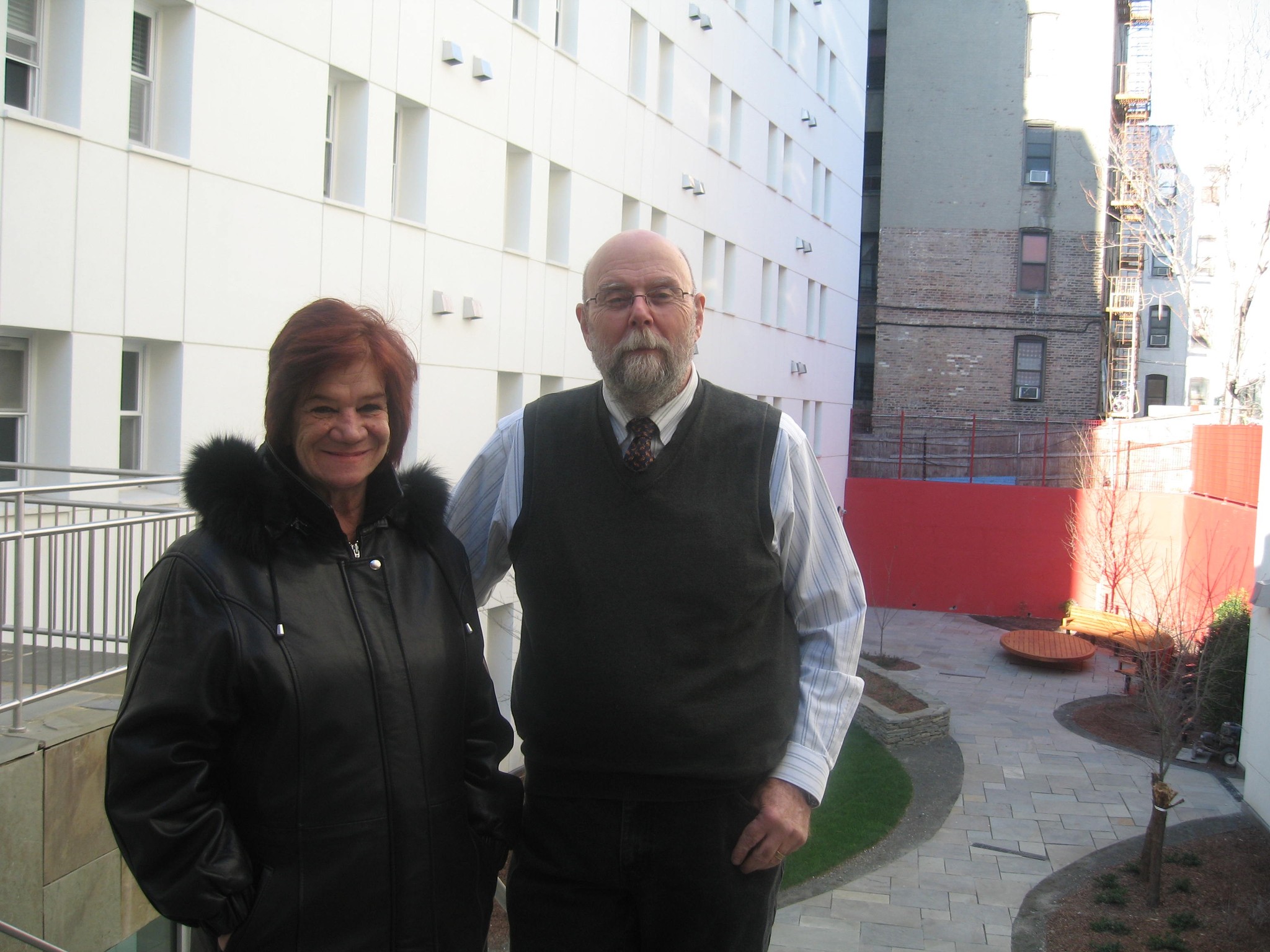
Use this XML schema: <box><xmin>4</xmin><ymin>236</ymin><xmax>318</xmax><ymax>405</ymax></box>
<box><xmin>775</xmin><ymin>852</ymin><xmax>786</xmax><ymax>861</ymax></box>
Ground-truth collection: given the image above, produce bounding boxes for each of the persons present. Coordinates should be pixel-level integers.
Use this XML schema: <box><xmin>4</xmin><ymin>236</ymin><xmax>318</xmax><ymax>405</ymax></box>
<box><xmin>439</xmin><ymin>228</ymin><xmax>866</xmax><ymax>952</ymax></box>
<box><xmin>104</xmin><ymin>298</ymin><xmax>518</xmax><ymax>952</ymax></box>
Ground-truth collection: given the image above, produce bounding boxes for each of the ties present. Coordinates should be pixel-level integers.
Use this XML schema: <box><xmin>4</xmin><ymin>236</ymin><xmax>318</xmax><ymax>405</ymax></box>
<box><xmin>624</xmin><ymin>416</ymin><xmax>657</xmax><ymax>473</ymax></box>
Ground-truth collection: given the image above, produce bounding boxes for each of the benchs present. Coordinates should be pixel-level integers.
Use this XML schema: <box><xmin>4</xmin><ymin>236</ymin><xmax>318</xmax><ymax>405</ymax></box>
<box><xmin>1059</xmin><ymin>605</ymin><xmax>1174</xmax><ymax>694</ymax></box>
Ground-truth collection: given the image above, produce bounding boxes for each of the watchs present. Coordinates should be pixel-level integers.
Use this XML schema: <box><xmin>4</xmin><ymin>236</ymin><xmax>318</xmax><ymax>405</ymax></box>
<box><xmin>803</xmin><ymin>790</ymin><xmax>818</xmax><ymax>807</ymax></box>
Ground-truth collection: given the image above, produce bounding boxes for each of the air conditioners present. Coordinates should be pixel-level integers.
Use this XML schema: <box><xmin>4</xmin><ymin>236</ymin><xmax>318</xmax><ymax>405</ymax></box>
<box><xmin>1157</xmin><ymin>268</ymin><xmax>1168</xmax><ymax>275</ymax></box>
<box><xmin>1018</xmin><ymin>387</ymin><xmax>1038</xmax><ymax>399</ymax></box>
<box><xmin>1150</xmin><ymin>335</ymin><xmax>1167</xmax><ymax>346</ymax></box>
<box><xmin>1112</xmin><ymin>397</ymin><xmax>1127</xmax><ymax>413</ymax></box>
<box><xmin>1029</xmin><ymin>170</ymin><xmax>1048</xmax><ymax>183</ymax></box>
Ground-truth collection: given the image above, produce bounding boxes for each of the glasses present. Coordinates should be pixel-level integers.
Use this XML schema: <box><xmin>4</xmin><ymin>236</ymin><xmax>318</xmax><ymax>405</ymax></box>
<box><xmin>585</xmin><ymin>286</ymin><xmax>691</xmax><ymax>311</ymax></box>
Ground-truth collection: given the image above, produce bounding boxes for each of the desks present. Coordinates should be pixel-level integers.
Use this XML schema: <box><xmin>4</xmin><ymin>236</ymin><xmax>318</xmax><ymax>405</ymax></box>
<box><xmin>1000</xmin><ymin>630</ymin><xmax>1095</xmax><ymax>671</ymax></box>
